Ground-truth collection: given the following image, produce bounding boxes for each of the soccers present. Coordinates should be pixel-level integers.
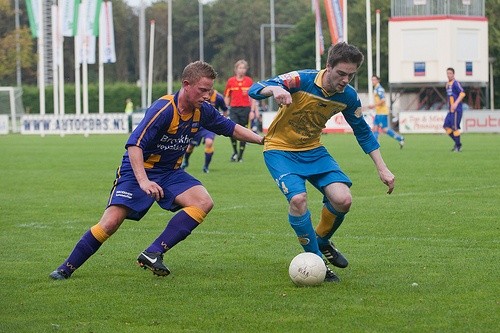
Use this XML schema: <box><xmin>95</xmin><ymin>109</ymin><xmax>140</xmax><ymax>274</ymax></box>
<box><xmin>289</xmin><ymin>251</ymin><xmax>327</xmax><ymax>286</ymax></box>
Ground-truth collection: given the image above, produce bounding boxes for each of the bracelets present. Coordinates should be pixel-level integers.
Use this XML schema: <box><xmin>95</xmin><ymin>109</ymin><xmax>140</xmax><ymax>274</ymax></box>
<box><xmin>250</xmin><ymin>110</ymin><xmax>255</xmax><ymax>113</ymax></box>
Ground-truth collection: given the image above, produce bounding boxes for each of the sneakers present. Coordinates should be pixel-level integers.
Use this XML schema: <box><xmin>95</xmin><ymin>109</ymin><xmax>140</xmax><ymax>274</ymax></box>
<box><xmin>50</xmin><ymin>269</ymin><xmax>67</xmax><ymax>280</ymax></box>
<box><xmin>323</xmin><ymin>259</ymin><xmax>341</xmax><ymax>284</ymax></box>
<box><xmin>318</xmin><ymin>241</ymin><xmax>348</xmax><ymax>269</ymax></box>
<box><xmin>137</xmin><ymin>250</ymin><xmax>170</xmax><ymax>277</ymax></box>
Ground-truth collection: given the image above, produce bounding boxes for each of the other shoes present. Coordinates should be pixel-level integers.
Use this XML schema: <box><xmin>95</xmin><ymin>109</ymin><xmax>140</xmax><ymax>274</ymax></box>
<box><xmin>231</xmin><ymin>151</ymin><xmax>238</xmax><ymax>161</ymax></box>
<box><xmin>238</xmin><ymin>156</ymin><xmax>242</xmax><ymax>162</ymax></box>
<box><xmin>181</xmin><ymin>162</ymin><xmax>188</xmax><ymax>169</ymax></box>
<box><xmin>453</xmin><ymin>143</ymin><xmax>463</xmax><ymax>154</ymax></box>
<box><xmin>400</xmin><ymin>137</ymin><xmax>405</xmax><ymax>148</ymax></box>
<box><xmin>204</xmin><ymin>168</ymin><xmax>208</xmax><ymax>173</ymax></box>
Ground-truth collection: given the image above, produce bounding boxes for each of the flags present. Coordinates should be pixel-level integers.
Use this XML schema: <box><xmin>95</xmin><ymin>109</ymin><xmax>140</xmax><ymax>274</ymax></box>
<box><xmin>25</xmin><ymin>0</ymin><xmax>116</xmax><ymax>65</ymax></box>
<box><xmin>310</xmin><ymin>0</ymin><xmax>344</xmax><ymax>56</ymax></box>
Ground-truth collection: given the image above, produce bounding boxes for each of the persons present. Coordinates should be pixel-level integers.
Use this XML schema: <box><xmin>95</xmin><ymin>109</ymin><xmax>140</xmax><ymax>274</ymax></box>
<box><xmin>124</xmin><ymin>97</ymin><xmax>133</xmax><ymax>134</ymax></box>
<box><xmin>49</xmin><ymin>60</ymin><xmax>268</xmax><ymax>282</ymax></box>
<box><xmin>462</xmin><ymin>97</ymin><xmax>470</xmax><ymax>110</ymax></box>
<box><xmin>224</xmin><ymin>59</ymin><xmax>257</xmax><ymax>162</ymax></box>
<box><xmin>179</xmin><ymin>86</ymin><xmax>229</xmax><ymax>173</ymax></box>
<box><xmin>443</xmin><ymin>67</ymin><xmax>467</xmax><ymax>152</ymax></box>
<box><xmin>367</xmin><ymin>75</ymin><xmax>405</xmax><ymax>150</ymax></box>
<box><xmin>246</xmin><ymin>73</ymin><xmax>268</xmax><ymax>145</ymax></box>
<box><xmin>248</xmin><ymin>41</ymin><xmax>395</xmax><ymax>284</ymax></box>
